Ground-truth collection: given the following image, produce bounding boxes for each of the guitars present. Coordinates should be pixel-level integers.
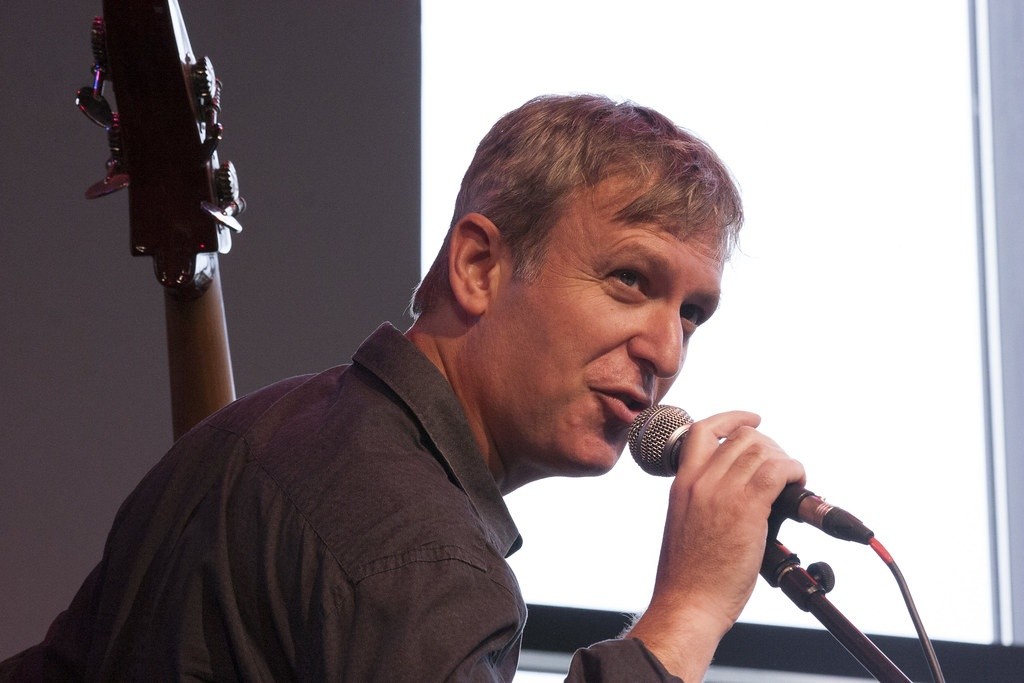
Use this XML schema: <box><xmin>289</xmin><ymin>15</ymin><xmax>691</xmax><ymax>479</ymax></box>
<box><xmin>73</xmin><ymin>0</ymin><xmax>246</xmax><ymax>444</ymax></box>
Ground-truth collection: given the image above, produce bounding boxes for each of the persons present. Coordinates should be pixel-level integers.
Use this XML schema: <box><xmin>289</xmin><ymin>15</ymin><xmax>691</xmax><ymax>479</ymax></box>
<box><xmin>0</xmin><ymin>96</ymin><xmax>807</xmax><ymax>683</ymax></box>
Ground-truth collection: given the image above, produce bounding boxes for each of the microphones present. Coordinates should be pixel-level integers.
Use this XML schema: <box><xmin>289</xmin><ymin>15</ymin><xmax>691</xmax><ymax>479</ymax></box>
<box><xmin>626</xmin><ymin>403</ymin><xmax>873</xmax><ymax>544</ymax></box>
